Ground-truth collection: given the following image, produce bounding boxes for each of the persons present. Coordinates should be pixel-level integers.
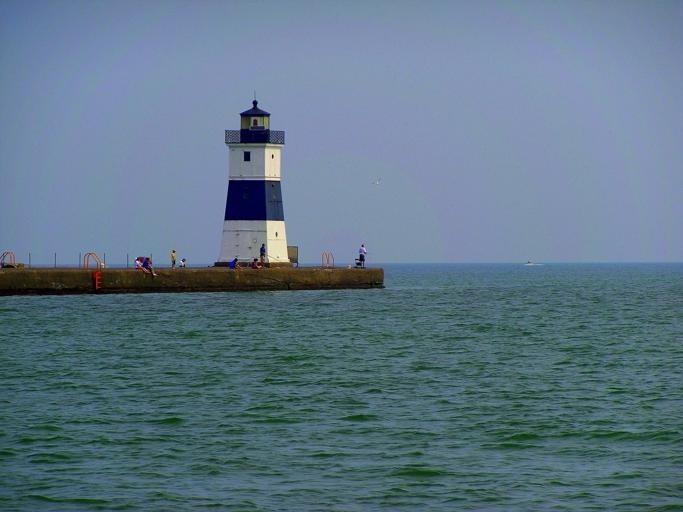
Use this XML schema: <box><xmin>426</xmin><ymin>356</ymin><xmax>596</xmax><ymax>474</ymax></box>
<box><xmin>359</xmin><ymin>244</ymin><xmax>366</xmax><ymax>268</ymax></box>
<box><xmin>135</xmin><ymin>256</ymin><xmax>151</xmax><ymax>274</ymax></box>
<box><xmin>252</xmin><ymin>259</ymin><xmax>262</xmax><ymax>269</ymax></box>
<box><xmin>259</xmin><ymin>243</ymin><xmax>265</xmax><ymax>268</ymax></box>
<box><xmin>143</xmin><ymin>258</ymin><xmax>159</xmax><ymax>277</ymax></box>
<box><xmin>179</xmin><ymin>259</ymin><xmax>185</xmax><ymax>269</ymax></box>
<box><xmin>230</xmin><ymin>258</ymin><xmax>240</xmax><ymax>268</ymax></box>
<box><xmin>171</xmin><ymin>250</ymin><xmax>176</xmax><ymax>270</ymax></box>
